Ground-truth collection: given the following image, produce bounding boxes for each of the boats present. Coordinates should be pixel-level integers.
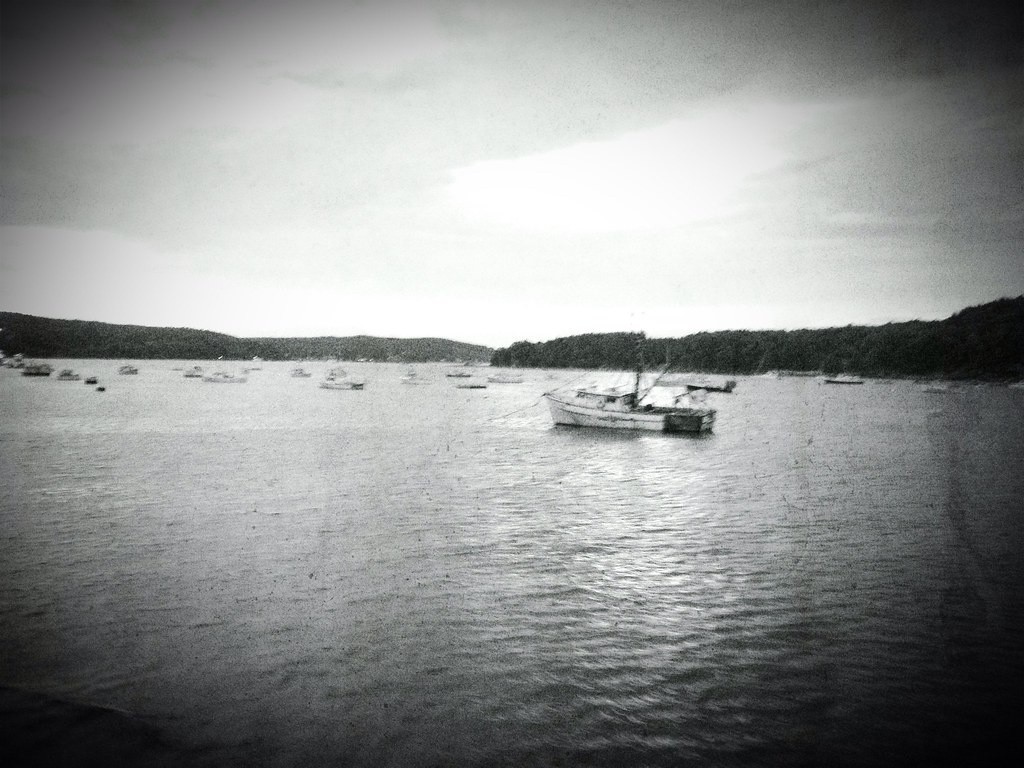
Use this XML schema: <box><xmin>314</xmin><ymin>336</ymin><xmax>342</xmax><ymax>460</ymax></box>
<box><xmin>402</xmin><ymin>366</ymin><xmax>428</xmax><ymax>385</ymax></box>
<box><xmin>824</xmin><ymin>377</ymin><xmax>866</xmax><ymax>385</ymax></box>
<box><xmin>486</xmin><ymin>372</ymin><xmax>524</xmax><ymax>384</ymax></box>
<box><xmin>19</xmin><ymin>363</ymin><xmax>54</xmax><ymax>375</ymax></box>
<box><xmin>118</xmin><ymin>364</ymin><xmax>138</xmax><ymax>374</ymax></box>
<box><xmin>83</xmin><ymin>377</ymin><xmax>100</xmax><ymax>386</ymax></box>
<box><xmin>455</xmin><ymin>385</ymin><xmax>487</xmax><ymax>390</ymax></box>
<box><xmin>290</xmin><ymin>368</ymin><xmax>313</xmax><ymax>377</ymax></box>
<box><xmin>58</xmin><ymin>369</ymin><xmax>80</xmax><ymax>382</ymax></box>
<box><xmin>202</xmin><ymin>369</ymin><xmax>249</xmax><ymax>386</ymax></box>
<box><xmin>0</xmin><ymin>349</ymin><xmax>26</xmax><ymax>370</ymax></box>
<box><xmin>172</xmin><ymin>366</ymin><xmax>205</xmax><ymax>379</ymax></box>
<box><xmin>545</xmin><ymin>336</ymin><xmax>718</xmax><ymax>434</ymax></box>
<box><xmin>682</xmin><ymin>379</ymin><xmax>738</xmax><ymax>393</ymax></box>
<box><xmin>318</xmin><ymin>375</ymin><xmax>366</xmax><ymax>392</ymax></box>
<box><xmin>447</xmin><ymin>371</ymin><xmax>475</xmax><ymax>379</ymax></box>
